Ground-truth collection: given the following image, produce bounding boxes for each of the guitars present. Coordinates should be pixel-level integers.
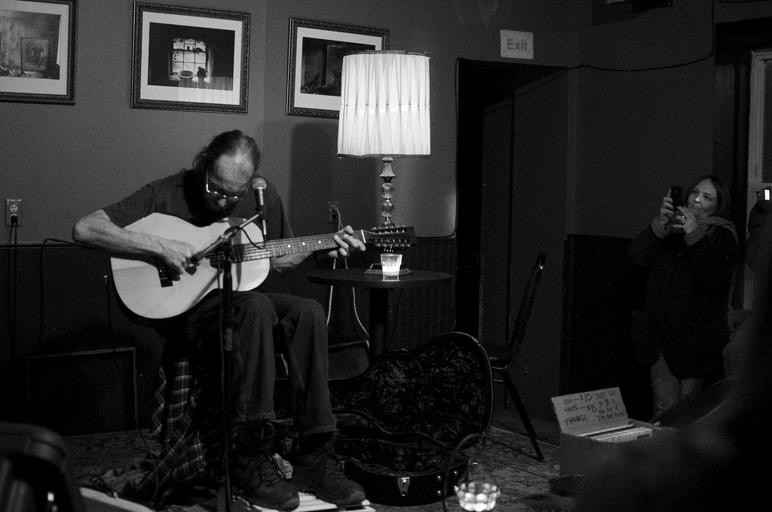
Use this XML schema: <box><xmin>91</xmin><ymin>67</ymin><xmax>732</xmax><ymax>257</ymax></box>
<box><xmin>110</xmin><ymin>212</ymin><xmax>418</xmax><ymax>320</ymax></box>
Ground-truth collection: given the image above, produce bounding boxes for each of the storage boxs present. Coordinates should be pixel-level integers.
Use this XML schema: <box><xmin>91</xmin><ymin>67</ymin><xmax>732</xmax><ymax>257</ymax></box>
<box><xmin>550</xmin><ymin>386</ymin><xmax>656</xmax><ymax>476</ymax></box>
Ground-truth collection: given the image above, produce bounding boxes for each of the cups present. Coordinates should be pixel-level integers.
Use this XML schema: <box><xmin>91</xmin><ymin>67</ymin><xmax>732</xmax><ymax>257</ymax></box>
<box><xmin>380</xmin><ymin>252</ymin><xmax>404</xmax><ymax>282</ymax></box>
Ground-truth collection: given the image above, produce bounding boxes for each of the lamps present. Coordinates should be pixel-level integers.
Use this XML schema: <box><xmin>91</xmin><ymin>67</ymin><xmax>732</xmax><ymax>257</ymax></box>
<box><xmin>337</xmin><ymin>50</ymin><xmax>431</xmax><ymax>276</ymax></box>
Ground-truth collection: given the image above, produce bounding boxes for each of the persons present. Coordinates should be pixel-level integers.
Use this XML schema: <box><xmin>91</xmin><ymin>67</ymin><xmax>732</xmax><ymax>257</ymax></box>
<box><xmin>630</xmin><ymin>174</ymin><xmax>740</xmax><ymax>416</ymax></box>
<box><xmin>73</xmin><ymin>130</ymin><xmax>367</xmax><ymax>512</ymax></box>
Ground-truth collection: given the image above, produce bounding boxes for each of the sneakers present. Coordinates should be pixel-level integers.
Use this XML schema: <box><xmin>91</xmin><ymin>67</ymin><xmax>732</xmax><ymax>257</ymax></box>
<box><xmin>291</xmin><ymin>442</ymin><xmax>366</xmax><ymax>506</ymax></box>
<box><xmin>229</xmin><ymin>448</ymin><xmax>298</xmax><ymax>512</ymax></box>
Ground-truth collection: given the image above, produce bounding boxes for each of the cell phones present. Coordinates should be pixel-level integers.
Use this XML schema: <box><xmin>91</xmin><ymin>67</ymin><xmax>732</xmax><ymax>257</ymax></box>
<box><xmin>671</xmin><ymin>183</ymin><xmax>684</xmax><ymax>212</ymax></box>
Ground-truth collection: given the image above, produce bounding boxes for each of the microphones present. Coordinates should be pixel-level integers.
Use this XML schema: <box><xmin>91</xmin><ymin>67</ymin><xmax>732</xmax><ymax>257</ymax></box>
<box><xmin>252</xmin><ymin>176</ymin><xmax>267</xmax><ymax>244</ymax></box>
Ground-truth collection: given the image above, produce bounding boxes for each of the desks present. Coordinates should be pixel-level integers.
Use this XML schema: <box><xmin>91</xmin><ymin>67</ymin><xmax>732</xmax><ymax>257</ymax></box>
<box><xmin>306</xmin><ymin>270</ymin><xmax>451</xmax><ymax>381</ymax></box>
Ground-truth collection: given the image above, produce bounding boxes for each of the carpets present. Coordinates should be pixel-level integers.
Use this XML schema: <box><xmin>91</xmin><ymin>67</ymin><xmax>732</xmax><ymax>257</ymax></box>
<box><xmin>59</xmin><ymin>422</ymin><xmax>559</xmax><ymax>512</ymax></box>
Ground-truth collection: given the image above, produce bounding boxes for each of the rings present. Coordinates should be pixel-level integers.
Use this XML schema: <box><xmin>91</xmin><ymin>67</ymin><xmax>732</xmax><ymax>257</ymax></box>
<box><xmin>679</xmin><ymin>216</ymin><xmax>685</xmax><ymax>222</ymax></box>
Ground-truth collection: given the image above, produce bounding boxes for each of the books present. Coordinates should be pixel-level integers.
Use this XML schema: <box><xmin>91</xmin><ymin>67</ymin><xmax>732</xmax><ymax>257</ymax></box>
<box><xmin>575</xmin><ymin>425</ymin><xmax>656</xmax><ymax>442</ymax></box>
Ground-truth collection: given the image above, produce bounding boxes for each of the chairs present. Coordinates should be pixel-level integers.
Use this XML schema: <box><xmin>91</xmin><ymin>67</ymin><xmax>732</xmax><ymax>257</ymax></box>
<box><xmin>480</xmin><ymin>249</ymin><xmax>545</xmax><ymax>461</ymax></box>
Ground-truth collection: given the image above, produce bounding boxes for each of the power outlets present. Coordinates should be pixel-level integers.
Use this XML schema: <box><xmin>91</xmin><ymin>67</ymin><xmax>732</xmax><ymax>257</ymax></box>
<box><xmin>326</xmin><ymin>200</ymin><xmax>343</xmax><ymax>224</ymax></box>
<box><xmin>5</xmin><ymin>198</ymin><xmax>22</xmax><ymax>227</ymax></box>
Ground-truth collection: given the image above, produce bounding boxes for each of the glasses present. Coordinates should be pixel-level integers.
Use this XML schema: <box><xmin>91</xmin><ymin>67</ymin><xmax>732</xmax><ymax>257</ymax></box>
<box><xmin>202</xmin><ymin>171</ymin><xmax>249</xmax><ymax>205</ymax></box>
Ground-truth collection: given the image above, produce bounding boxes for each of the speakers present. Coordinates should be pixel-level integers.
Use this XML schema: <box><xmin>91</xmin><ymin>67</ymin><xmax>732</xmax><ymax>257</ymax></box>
<box><xmin>23</xmin><ymin>346</ymin><xmax>139</xmax><ymax>438</ymax></box>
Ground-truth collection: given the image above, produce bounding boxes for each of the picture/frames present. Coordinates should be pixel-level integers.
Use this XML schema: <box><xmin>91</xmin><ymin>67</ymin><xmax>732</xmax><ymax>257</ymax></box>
<box><xmin>0</xmin><ymin>0</ymin><xmax>78</xmax><ymax>105</ymax></box>
<box><xmin>130</xmin><ymin>1</ymin><xmax>251</xmax><ymax>113</ymax></box>
<box><xmin>286</xmin><ymin>17</ymin><xmax>390</xmax><ymax>119</ymax></box>
